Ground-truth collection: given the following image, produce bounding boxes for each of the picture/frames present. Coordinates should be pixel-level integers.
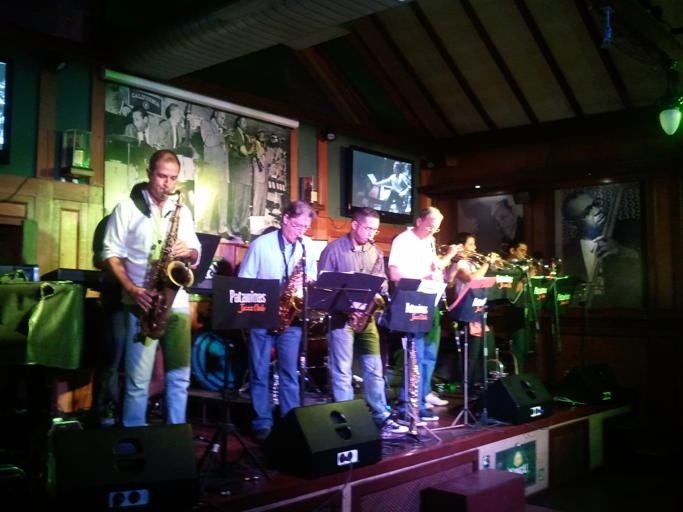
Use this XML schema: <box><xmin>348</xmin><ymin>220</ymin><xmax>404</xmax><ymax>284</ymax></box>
<box><xmin>451</xmin><ymin>187</ymin><xmax>533</xmax><ymax>254</ymax></box>
<box><xmin>546</xmin><ymin>174</ymin><xmax>657</xmax><ymax>323</ymax></box>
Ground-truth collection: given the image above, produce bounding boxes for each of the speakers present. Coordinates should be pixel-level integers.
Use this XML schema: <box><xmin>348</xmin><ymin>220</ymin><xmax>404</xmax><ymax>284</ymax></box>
<box><xmin>471</xmin><ymin>372</ymin><xmax>553</xmax><ymax>425</ymax></box>
<box><xmin>52</xmin><ymin>424</ymin><xmax>198</xmax><ymax>512</ymax></box>
<box><xmin>554</xmin><ymin>367</ymin><xmax>620</xmax><ymax>408</ymax></box>
<box><xmin>261</xmin><ymin>398</ymin><xmax>382</xmax><ymax>480</ymax></box>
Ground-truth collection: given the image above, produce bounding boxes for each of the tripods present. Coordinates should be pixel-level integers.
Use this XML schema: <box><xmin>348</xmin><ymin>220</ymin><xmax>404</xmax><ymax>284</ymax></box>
<box><xmin>450</xmin><ymin>323</ymin><xmax>480</xmax><ymax>430</ymax></box>
<box><xmin>379</xmin><ymin>334</ymin><xmax>442</xmax><ymax>443</ymax></box>
<box><xmin>196</xmin><ymin>328</ymin><xmax>273</xmax><ymax>497</ymax></box>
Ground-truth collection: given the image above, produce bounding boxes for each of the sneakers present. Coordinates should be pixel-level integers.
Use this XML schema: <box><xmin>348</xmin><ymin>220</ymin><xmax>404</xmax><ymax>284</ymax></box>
<box><xmin>381</xmin><ymin>391</ymin><xmax>449</xmax><ymax>433</ymax></box>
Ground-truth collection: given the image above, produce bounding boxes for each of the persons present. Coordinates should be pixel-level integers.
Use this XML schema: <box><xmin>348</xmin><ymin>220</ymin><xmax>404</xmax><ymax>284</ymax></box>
<box><xmin>452</xmin><ymin>232</ymin><xmax>490</xmax><ymax>395</ymax></box>
<box><xmin>387</xmin><ymin>206</ymin><xmax>445</xmax><ymax>426</ymax></box>
<box><xmin>561</xmin><ymin>190</ymin><xmax>642</xmax><ymax>307</ymax></box>
<box><xmin>316</xmin><ymin>207</ymin><xmax>410</xmax><ymax>433</ymax></box>
<box><xmin>124</xmin><ymin>102</ymin><xmax>287</xmax><ymax>241</ymax></box>
<box><xmin>504</xmin><ymin>238</ymin><xmax>537</xmax><ymax>371</ymax></box>
<box><xmin>237</xmin><ymin>199</ymin><xmax>317</xmax><ymax>440</ymax></box>
<box><xmin>371</xmin><ymin>161</ymin><xmax>412</xmax><ymax>213</ymax></box>
<box><xmin>101</xmin><ymin>147</ymin><xmax>203</xmax><ymax>428</ymax></box>
<box><xmin>419</xmin><ymin>241</ymin><xmax>465</xmax><ymax>410</ymax></box>
<box><xmin>491</xmin><ymin>199</ymin><xmax>523</xmax><ymax>238</ymax></box>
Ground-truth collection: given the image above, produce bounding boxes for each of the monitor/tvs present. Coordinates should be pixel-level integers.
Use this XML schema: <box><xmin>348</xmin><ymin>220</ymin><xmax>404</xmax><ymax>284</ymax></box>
<box><xmin>346</xmin><ymin>144</ymin><xmax>415</xmax><ymax>223</ymax></box>
<box><xmin>0</xmin><ymin>55</ymin><xmax>11</xmax><ymax>164</ymax></box>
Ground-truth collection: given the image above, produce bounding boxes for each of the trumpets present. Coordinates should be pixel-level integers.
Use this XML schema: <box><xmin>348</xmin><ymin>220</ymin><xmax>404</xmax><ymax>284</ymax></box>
<box><xmin>435</xmin><ymin>243</ymin><xmax>562</xmax><ymax>273</ymax></box>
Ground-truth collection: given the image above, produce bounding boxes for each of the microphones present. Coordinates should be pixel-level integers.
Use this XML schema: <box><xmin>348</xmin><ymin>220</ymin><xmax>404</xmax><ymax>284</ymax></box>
<box><xmin>550</xmin><ymin>257</ymin><xmax>560</xmax><ymax>261</ymax></box>
<box><xmin>511</xmin><ymin>259</ymin><xmax>531</xmax><ymax>265</ymax></box>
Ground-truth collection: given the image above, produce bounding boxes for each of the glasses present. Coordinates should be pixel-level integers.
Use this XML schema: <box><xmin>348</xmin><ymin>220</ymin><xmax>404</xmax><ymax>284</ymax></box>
<box><xmin>422</xmin><ymin>223</ymin><xmax>441</xmax><ymax>233</ymax></box>
<box><xmin>579</xmin><ymin>198</ymin><xmax>604</xmax><ymax>218</ymax></box>
<box><xmin>358</xmin><ymin>224</ymin><xmax>380</xmax><ymax>236</ymax></box>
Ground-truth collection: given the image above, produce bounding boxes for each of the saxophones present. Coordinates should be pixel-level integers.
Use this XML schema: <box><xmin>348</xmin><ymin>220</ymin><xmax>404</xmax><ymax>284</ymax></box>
<box><xmin>351</xmin><ymin>239</ymin><xmax>386</xmax><ymax>334</ymax></box>
<box><xmin>273</xmin><ymin>238</ymin><xmax>307</xmax><ymax>334</ymax></box>
<box><xmin>140</xmin><ymin>190</ymin><xmax>194</xmax><ymax>338</ymax></box>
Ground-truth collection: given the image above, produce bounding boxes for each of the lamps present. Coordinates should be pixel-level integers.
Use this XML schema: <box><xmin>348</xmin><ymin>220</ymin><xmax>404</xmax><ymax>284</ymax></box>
<box><xmin>324</xmin><ymin>125</ymin><xmax>337</xmax><ymax>140</ymax></box>
<box><xmin>657</xmin><ymin>94</ymin><xmax>682</xmax><ymax>138</ymax></box>
